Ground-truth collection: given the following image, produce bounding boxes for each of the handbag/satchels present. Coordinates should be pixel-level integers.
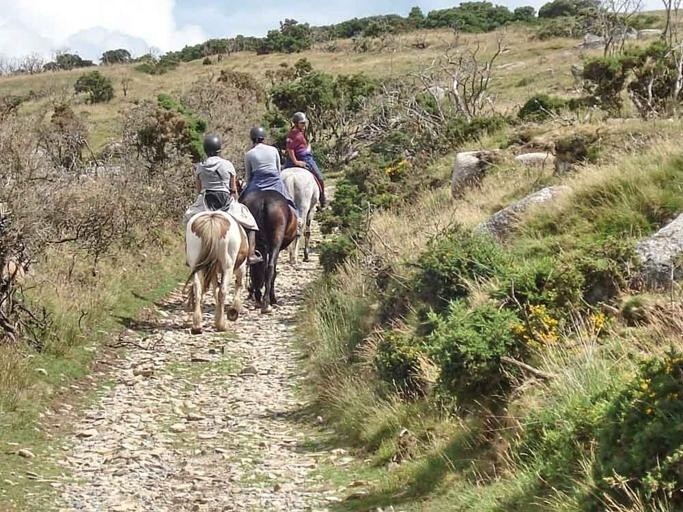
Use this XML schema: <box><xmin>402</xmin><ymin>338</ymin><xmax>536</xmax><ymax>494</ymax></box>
<box><xmin>203</xmin><ymin>188</ymin><xmax>232</xmax><ymax>211</ymax></box>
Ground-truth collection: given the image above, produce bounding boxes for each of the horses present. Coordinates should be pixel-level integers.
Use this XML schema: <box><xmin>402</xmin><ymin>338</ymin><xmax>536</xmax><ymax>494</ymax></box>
<box><xmin>240</xmin><ymin>189</ymin><xmax>298</xmax><ymax>314</ymax></box>
<box><xmin>183</xmin><ymin>209</ymin><xmax>249</xmax><ymax>335</ymax></box>
<box><xmin>279</xmin><ymin>167</ymin><xmax>320</xmax><ymax>265</ymax></box>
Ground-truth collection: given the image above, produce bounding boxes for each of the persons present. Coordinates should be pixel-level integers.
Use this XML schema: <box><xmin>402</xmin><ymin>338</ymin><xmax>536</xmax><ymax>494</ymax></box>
<box><xmin>281</xmin><ymin>111</ymin><xmax>328</xmax><ymax>210</ymax></box>
<box><xmin>181</xmin><ymin>135</ymin><xmax>262</xmax><ymax>268</ymax></box>
<box><xmin>240</xmin><ymin>125</ymin><xmax>303</xmax><ymax>239</ymax></box>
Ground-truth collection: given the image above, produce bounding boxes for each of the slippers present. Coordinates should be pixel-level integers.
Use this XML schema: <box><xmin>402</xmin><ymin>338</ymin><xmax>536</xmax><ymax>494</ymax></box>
<box><xmin>247</xmin><ymin>256</ymin><xmax>265</xmax><ymax>265</ymax></box>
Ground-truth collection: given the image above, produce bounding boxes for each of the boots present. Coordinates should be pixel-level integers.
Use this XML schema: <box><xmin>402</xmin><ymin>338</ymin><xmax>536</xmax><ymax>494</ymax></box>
<box><xmin>317</xmin><ymin>181</ymin><xmax>331</xmax><ymax>209</ymax></box>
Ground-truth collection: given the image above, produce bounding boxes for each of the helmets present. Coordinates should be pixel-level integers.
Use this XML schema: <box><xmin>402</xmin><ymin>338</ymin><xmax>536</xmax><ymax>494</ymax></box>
<box><xmin>201</xmin><ymin>133</ymin><xmax>223</xmax><ymax>154</ymax></box>
<box><xmin>290</xmin><ymin>111</ymin><xmax>308</xmax><ymax>126</ymax></box>
<box><xmin>249</xmin><ymin>126</ymin><xmax>267</xmax><ymax>142</ymax></box>
